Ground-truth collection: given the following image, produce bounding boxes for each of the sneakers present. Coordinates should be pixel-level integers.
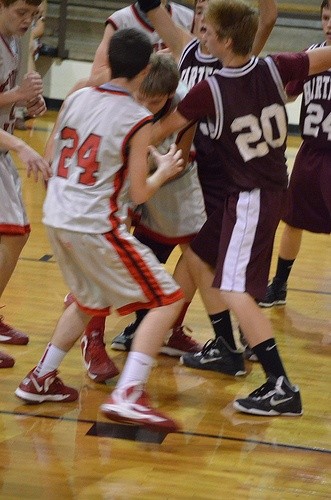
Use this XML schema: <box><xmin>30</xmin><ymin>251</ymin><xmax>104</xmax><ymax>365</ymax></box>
<box><xmin>233</xmin><ymin>376</ymin><xmax>303</xmax><ymax>415</ymax></box>
<box><xmin>258</xmin><ymin>278</ymin><xmax>287</xmax><ymax>307</ymax></box>
<box><xmin>0</xmin><ymin>352</ymin><xmax>15</xmax><ymax>368</ymax></box>
<box><xmin>0</xmin><ymin>314</ymin><xmax>29</xmax><ymax>345</ymax></box>
<box><xmin>80</xmin><ymin>329</ymin><xmax>120</xmax><ymax>383</ymax></box>
<box><xmin>157</xmin><ymin>325</ymin><xmax>203</xmax><ymax>356</ymax></box>
<box><xmin>100</xmin><ymin>383</ymin><xmax>179</xmax><ymax>432</ymax></box>
<box><xmin>15</xmin><ymin>365</ymin><xmax>78</xmax><ymax>403</ymax></box>
<box><xmin>111</xmin><ymin>320</ymin><xmax>139</xmax><ymax>350</ymax></box>
<box><xmin>180</xmin><ymin>336</ymin><xmax>247</xmax><ymax>376</ymax></box>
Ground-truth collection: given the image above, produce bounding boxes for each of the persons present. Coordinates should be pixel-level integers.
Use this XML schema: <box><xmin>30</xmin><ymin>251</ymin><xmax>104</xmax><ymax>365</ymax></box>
<box><xmin>13</xmin><ymin>28</ymin><xmax>185</xmax><ymax>433</ymax></box>
<box><xmin>258</xmin><ymin>0</ymin><xmax>331</xmax><ymax>308</ymax></box>
<box><xmin>148</xmin><ymin>0</ymin><xmax>331</xmax><ymax>416</ymax></box>
<box><xmin>0</xmin><ymin>0</ymin><xmax>53</xmax><ymax>368</ymax></box>
<box><xmin>65</xmin><ymin>0</ymin><xmax>278</xmax><ymax>384</ymax></box>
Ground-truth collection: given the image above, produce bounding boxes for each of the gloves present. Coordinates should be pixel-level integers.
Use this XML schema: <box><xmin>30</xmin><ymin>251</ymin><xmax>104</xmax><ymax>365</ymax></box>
<box><xmin>138</xmin><ymin>0</ymin><xmax>161</xmax><ymax>13</ymax></box>
